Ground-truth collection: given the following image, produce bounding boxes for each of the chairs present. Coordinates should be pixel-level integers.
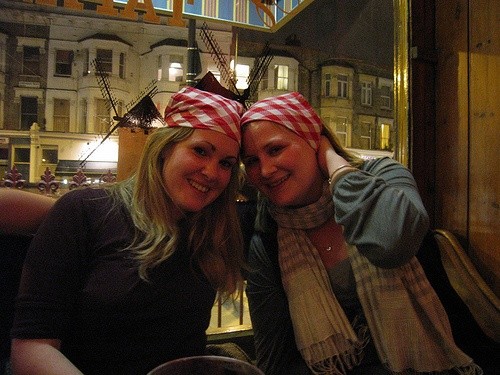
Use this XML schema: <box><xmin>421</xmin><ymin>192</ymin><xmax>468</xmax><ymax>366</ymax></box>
<box><xmin>415</xmin><ymin>226</ymin><xmax>500</xmax><ymax>375</ymax></box>
<box><xmin>0</xmin><ymin>187</ymin><xmax>56</xmax><ymax>324</ymax></box>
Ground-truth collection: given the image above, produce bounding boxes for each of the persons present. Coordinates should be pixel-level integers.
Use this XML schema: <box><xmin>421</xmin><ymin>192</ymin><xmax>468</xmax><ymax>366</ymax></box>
<box><xmin>5</xmin><ymin>83</ymin><xmax>248</xmax><ymax>373</ymax></box>
<box><xmin>237</xmin><ymin>92</ymin><xmax>500</xmax><ymax>375</ymax></box>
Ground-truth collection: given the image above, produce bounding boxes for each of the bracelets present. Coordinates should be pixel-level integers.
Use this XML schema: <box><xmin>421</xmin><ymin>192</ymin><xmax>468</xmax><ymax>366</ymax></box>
<box><xmin>331</xmin><ymin>165</ymin><xmax>352</xmax><ymax>187</ymax></box>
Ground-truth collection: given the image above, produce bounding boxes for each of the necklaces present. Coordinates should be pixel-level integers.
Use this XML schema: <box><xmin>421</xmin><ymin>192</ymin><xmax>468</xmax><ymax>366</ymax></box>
<box><xmin>316</xmin><ymin>230</ymin><xmax>342</xmax><ymax>252</ymax></box>
